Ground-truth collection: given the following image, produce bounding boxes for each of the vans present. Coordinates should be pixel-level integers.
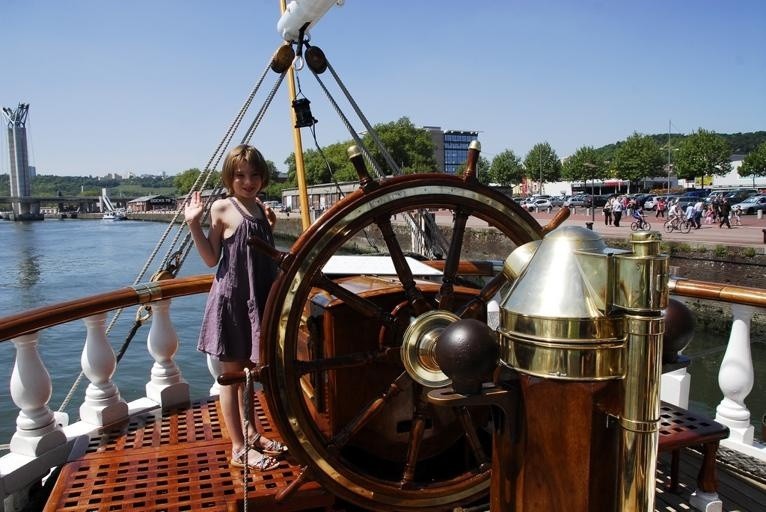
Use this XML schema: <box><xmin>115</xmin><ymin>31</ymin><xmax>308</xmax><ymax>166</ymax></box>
<box><xmin>705</xmin><ymin>189</ymin><xmax>766</xmax><ymax>215</ymax></box>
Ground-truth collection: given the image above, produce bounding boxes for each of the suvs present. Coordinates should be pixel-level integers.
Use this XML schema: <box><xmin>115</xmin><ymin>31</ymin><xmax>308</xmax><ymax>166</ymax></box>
<box><xmin>636</xmin><ymin>191</ymin><xmax>708</xmax><ymax>212</ymax></box>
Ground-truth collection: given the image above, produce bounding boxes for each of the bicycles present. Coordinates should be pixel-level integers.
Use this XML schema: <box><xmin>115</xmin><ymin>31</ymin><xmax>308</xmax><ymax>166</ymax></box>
<box><xmin>664</xmin><ymin>213</ymin><xmax>691</xmax><ymax>234</ymax></box>
<box><xmin>631</xmin><ymin>215</ymin><xmax>651</xmax><ymax>231</ymax></box>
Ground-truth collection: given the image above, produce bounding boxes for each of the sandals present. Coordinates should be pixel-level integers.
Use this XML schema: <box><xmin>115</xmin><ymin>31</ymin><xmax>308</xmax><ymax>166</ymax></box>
<box><xmin>231</xmin><ymin>445</ymin><xmax>280</xmax><ymax>470</ymax></box>
<box><xmin>248</xmin><ymin>432</ymin><xmax>288</xmax><ymax>456</ymax></box>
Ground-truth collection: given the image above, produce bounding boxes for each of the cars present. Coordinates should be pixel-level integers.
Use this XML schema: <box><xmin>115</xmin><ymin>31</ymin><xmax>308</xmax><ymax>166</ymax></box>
<box><xmin>520</xmin><ymin>193</ymin><xmax>607</xmax><ymax>212</ymax></box>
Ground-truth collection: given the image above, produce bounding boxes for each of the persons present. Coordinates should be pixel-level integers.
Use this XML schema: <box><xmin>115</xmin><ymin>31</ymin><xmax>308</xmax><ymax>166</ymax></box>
<box><xmin>602</xmin><ymin>194</ymin><xmax>743</xmax><ymax>229</ymax></box>
<box><xmin>186</xmin><ymin>144</ymin><xmax>291</xmax><ymax>471</ymax></box>
